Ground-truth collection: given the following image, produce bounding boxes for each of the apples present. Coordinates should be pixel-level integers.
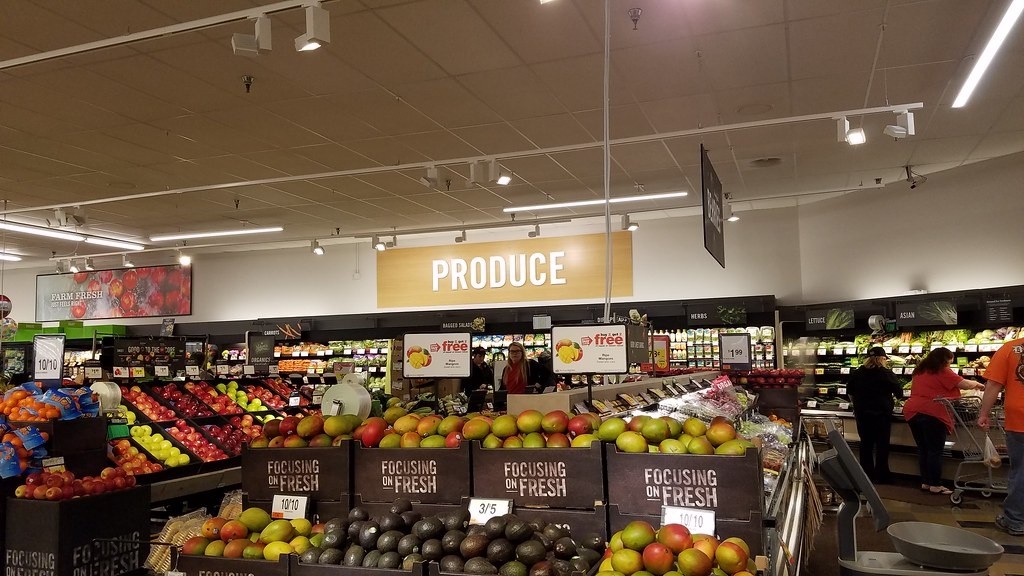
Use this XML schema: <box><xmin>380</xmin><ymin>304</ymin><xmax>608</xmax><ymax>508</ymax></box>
<box><xmin>70</xmin><ymin>267</ymin><xmax>190</xmax><ymax>317</ymax></box>
<box><xmin>108</xmin><ymin>377</ymin><xmax>320</xmax><ymax>475</ymax></box>
<box><xmin>722</xmin><ymin>366</ymin><xmax>805</xmax><ymax>389</ymax></box>
<box><xmin>17</xmin><ymin>467</ymin><xmax>135</xmax><ymax>500</ymax></box>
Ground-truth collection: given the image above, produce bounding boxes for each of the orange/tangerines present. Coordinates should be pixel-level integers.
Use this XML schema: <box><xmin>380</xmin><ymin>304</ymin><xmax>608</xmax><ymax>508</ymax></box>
<box><xmin>0</xmin><ymin>389</ymin><xmax>60</xmax><ymax>471</ymax></box>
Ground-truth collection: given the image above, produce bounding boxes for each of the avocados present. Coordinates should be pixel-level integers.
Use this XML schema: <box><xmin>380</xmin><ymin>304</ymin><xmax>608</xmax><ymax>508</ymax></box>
<box><xmin>299</xmin><ymin>498</ymin><xmax>604</xmax><ymax>576</ymax></box>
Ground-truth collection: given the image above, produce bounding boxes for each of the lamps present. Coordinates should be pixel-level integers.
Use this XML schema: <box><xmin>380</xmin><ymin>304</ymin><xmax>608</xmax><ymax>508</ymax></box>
<box><xmin>832</xmin><ymin>115</ymin><xmax>866</xmax><ymax>145</ymax></box>
<box><xmin>232</xmin><ymin>13</ymin><xmax>273</xmax><ymax>55</ymax></box>
<box><xmin>486</xmin><ymin>159</ymin><xmax>512</xmax><ymax>185</ymax></box>
<box><xmin>455</xmin><ymin>229</ymin><xmax>467</xmax><ymax>243</ymax></box>
<box><xmin>465</xmin><ymin>160</ymin><xmax>483</xmax><ymax>190</ymax></box>
<box><xmin>420</xmin><ymin>165</ymin><xmax>442</xmax><ymax>187</ymax></box>
<box><xmin>370</xmin><ymin>234</ymin><xmax>386</xmax><ymax>251</ymax></box>
<box><xmin>724</xmin><ymin>200</ymin><xmax>739</xmax><ymax>224</ymax></box>
<box><xmin>175</xmin><ymin>249</ymin><xmax>191</xmax><ymax>267</ymax></box>
<box><xmin>294</xmin><ymin>0</ymin><xmax>331</xmax><ymax>51</ymax></box>
<box><xmin>311</xmin><ymin>238</ymin><xmax>325</xmax><ymax>255</ymax></box>
<box><xmin>386</xmin><ymin>235</ymin><xmax>397</xmax><ymax>247</ymax></box>
<box><xmin>620</xmin><ymin>212</ymin><xmax>639</xmax><ymax>231</ymax></box>
<box><xmin>55</xmin><ymin>257</ymin><xmax>95</xmax><ymax>275</ymax></box>
<box><xmin>45</xmin><ymin>206</ymin><xmax>89</xmax><ymax>229</ymax></box>
<box><xmin>883</xmin><ymin>110</ymin><xmax>916</xmax><ymax>138</ymax></box>
<box><xmin>528</xmin><ymin>224</ymin><xmax>541</xmax><ymax>237</ymax></box>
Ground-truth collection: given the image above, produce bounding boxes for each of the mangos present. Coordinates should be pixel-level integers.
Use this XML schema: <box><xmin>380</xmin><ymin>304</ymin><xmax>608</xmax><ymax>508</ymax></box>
<box><xmin>594</xmin><ymin>521</ymin><xmax>758</xmax><ymax>576</ymax></box>
<box><xmin>250</xmin><ymin>406</ymin><xmax>756</xmax><ymax>456</ymax></box>
<box><xmin>407</xmin><ymin>345</ymin><xmax>432</xmax><ymax>369</ymax></box>
<box><xmin>556</xmin><ymin>339</ymin><xmax>583</xmax><ymax>363</ymax></box>
<box><xmin>181</xmin><ymin>507</ymin><xmax>329</xmax><ymax>561</ymax></box>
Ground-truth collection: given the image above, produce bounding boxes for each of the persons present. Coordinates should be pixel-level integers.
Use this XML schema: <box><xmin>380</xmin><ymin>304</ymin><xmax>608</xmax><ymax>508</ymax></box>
<box><xmin>978</xmin><ymin>337</ymin><xmax>1024</xmax><ymax>536</ymax></box>
<box><xmin>498</xmin><ymin>342</ymin><xmax>551</xmax><ymax>405</ymax></box>
<box><xmin>904</xmin><ymin>347</ymin><xmax>985</xmax><ymax>493</ymax></box>
<box><xmin>846</xmin><ymin>347</ymin><xmax>903</xmax><ymax>485</ymax></box>
<box><xmin>465</xmin><ymin>347</ymin><xmax>495</xmax><ymax>398</ymax></box>
<box><xmin>538</xmin><ymin>352</ymin><xmax>557</xmax><ymax>394</ymax></box>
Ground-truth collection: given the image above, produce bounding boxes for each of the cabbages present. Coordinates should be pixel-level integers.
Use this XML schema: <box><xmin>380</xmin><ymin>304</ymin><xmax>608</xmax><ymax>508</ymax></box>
<box><xmin>969</xmin><ymin>329</ymin><xmax>999</xmax><ymax>344</ymax></box>
<box><xmin>368</xmin><ymin>376</ymin><xmax>386</xmax><ymax>390</ymax></box>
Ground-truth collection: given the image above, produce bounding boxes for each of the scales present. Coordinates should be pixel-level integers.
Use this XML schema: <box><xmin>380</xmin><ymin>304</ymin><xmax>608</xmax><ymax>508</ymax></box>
<box><xmin>817</xmin><ymin>418</ymin><xmax>1004</xmax><ymax>576</ymax></box>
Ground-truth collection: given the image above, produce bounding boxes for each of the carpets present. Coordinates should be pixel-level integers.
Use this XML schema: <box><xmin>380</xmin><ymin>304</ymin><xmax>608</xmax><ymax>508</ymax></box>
<box><xmin>798</xmin><ymin>511</ymin><xmax>920</xmax><ymax>576</ymax></box>
<box><xmin>860</xmin><ymin>472</ymin><xmax>975</xmax><ymax>507</ymax></box>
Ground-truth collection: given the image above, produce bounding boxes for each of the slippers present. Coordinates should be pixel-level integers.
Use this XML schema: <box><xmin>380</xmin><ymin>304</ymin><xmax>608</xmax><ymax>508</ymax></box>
<box><xmin>920</xmin><ymin>485</ymin><xmax>931</xmax><ymax>491</ymax></box>
<box><xmin>929</xmin><ymin>486</ymin><xmax>953</xmax><ymax>496</ymax></box>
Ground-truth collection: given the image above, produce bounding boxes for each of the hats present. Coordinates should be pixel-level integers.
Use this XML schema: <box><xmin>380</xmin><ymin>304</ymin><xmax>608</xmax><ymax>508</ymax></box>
<box><xmin>869</xmin><ymin>346</ymin><xmax>889</xmax><ymax>360</ymax></box>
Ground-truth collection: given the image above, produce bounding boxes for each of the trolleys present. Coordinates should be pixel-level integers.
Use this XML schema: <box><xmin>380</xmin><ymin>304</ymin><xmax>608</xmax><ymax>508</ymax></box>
<box><xmin>935</xmin><ymin>395</ymin><xmax>1011</xmax><ymax>504</ymax></box>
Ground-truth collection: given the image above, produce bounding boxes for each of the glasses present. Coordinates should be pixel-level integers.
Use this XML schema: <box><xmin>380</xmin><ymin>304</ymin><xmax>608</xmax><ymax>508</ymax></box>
<box><xmin>508</xmin><ymin>349</ymin><xmax>522</xmax><ymax>354</ymax></box>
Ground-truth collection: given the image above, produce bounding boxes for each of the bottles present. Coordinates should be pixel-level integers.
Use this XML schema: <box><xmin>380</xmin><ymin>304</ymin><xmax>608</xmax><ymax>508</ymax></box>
<box><xmin>648</xmin><ymin>329</ymin><xmax>687</xmax><ymax>343</ymax></box>
<box><xmin>670</xmin><ymin>349</ymin><xmax>687</xmax><ymax>360</ymax></box>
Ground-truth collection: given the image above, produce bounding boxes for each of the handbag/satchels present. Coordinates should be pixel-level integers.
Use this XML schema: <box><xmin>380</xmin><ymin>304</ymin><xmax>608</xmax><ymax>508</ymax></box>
<box><xmin>984</xmin><ymin>430</ymin><xmax>1001</xmax><ymax>468</ymax></box>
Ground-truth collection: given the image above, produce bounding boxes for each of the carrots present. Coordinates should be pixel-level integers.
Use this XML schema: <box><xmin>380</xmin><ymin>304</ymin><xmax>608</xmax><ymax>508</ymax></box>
<box><xmin>274</xmin><ymin>342</ymin><xmax>327</xmax><ymax>372</ymax></box>
<box><xmin>277</xmin><ymin>323</ymin><xmax>303</xmax><ymax>339</ymax></box>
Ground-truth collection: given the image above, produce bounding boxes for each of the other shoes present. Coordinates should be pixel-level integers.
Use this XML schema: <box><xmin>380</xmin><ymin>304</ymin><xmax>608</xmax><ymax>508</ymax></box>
<box><xmin>996</xmin><ymin>515</ymin><xmax>1024</xmax><ymax>536</ymax></box>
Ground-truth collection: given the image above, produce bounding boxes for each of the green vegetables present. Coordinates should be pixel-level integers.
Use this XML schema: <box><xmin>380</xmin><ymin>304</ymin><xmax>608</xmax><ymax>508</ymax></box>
<box><xmin>850</xmin><ymin>329</ymin><xmax>974</xmax><ymax>405</ymax></box>
<box><xmin>916</xmin><ymin>301</ymin><xmax>957</xmax><ymax>325</ymax></box>
<box><xmin>825</xmin><ymin>311</ymin><xmax>852</xmax><ymax>329</ymax></box>
<box><xmin>326</xmin><ymin>341</ymin><xmax>388</xmax><ymax>368</ymax></box>
